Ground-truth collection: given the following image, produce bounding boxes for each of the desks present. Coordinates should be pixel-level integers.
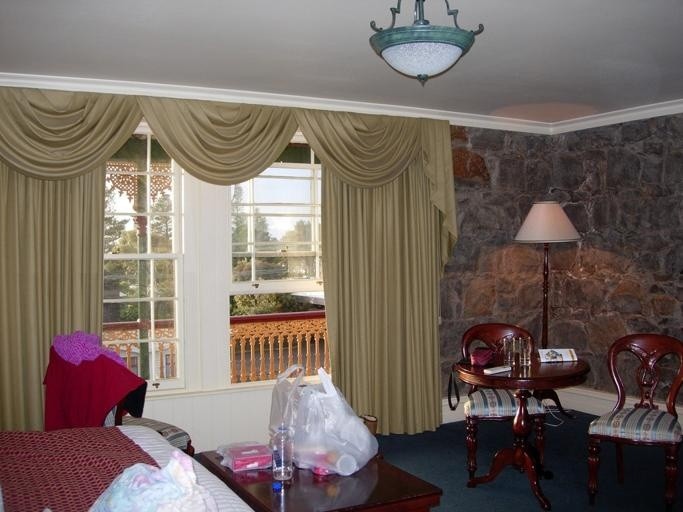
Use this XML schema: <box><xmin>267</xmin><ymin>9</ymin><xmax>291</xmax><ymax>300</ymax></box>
<box><xmin>194</xmin><ymin>444</ymin><xmax>443</xmax><ymax>512</ymax></box>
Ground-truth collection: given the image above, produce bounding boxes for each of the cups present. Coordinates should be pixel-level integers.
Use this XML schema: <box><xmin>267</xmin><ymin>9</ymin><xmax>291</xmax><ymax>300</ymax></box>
<box><xmin>519</xmin><ymin>336</ymin><xmax>532</xmax><ymax>366</ymax></box>
<box><xmin>502</xmin><ymin>336</ymin><xmax>515</xmax><ymax>366</ymax></box>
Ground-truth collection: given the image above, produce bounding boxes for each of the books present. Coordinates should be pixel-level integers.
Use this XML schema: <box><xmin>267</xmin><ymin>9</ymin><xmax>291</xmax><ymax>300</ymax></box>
<box><xmin>537</xmin><ymin>347</ymin><xmax>583</xmax><ymax>362</ymax></box>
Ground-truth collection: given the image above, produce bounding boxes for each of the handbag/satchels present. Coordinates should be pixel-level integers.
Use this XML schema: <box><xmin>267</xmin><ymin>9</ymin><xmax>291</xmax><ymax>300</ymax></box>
<box><xmin>447</xmin><ymin>345</ymin><xmax>501</xmax><ymax>410</ymax></box>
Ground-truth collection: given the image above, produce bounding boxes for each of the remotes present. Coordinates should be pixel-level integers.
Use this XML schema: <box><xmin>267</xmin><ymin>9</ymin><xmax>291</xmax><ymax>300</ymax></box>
<box><xmin>482</xmin><ymin>365</ymin><xmax>513</xmax><ymax>377</ymax></box>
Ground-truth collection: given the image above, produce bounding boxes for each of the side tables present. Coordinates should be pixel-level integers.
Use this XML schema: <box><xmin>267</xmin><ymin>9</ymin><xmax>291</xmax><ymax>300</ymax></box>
<box><xmin>453</xmin><ymin>352</ymin><xmax>592</xmax><ymax>512</ymax></box>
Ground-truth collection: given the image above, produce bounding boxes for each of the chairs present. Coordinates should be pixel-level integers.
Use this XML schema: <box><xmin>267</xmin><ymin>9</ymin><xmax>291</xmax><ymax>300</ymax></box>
<box><xmin>586</xmin><ymin>332</ymin><xmax>683</xmax><ymax>509</ymax></box>
<box><xmin>42</xmin><ymin>342</ymin><xmax>195</xmax><ymax>458</ymax></box>
<box><xmin>460</xmin><ymin>322</ymin><xmax>547</xmax><ymax>479</ymax></box>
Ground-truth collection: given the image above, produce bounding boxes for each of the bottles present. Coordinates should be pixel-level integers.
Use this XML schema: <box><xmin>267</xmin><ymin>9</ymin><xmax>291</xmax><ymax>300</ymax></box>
<box><xmin>272</xmin><ymin>423</ymin><xmax>293</xmax><ymax>480</ymax></box>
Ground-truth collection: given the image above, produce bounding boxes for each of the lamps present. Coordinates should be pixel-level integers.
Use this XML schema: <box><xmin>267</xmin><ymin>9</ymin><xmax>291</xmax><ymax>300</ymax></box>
<box><xmin>513</xmin><ymin>200</ymin><xmax>584</xmax><ymax>421</ymax></box>
<box><xmin>367</xmin><ymin>0</ymin><xmax>485</xmax><ymax>86</ymax></box>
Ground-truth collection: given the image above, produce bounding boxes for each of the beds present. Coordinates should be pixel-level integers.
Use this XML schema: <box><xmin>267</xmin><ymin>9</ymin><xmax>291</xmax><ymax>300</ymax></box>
<box><xmin>1</xmin><ymin>425</ymin><xmax>256</xmax><ymax>512</ymax></box>
